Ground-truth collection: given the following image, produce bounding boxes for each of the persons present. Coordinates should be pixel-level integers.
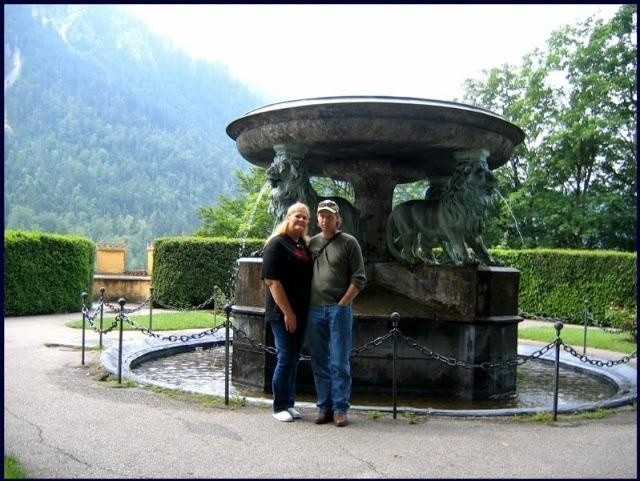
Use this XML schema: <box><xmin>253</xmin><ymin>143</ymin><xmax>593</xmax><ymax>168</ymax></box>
<box><xmin>260</xmin><ymin>202</ymin><xmax>315</xmax><ymax>424</ymax></box>
<box><xmin>302</xmin><ymin>199</ymin><xmax>368</xmax><ymax>428</ymax></box>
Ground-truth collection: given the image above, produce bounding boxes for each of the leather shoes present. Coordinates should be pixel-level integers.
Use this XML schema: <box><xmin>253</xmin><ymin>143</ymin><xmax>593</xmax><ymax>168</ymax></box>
<box><xmin>315</xmin><ymin>414</ymin><xmax>347</xmax><ymax>426</ymax></box>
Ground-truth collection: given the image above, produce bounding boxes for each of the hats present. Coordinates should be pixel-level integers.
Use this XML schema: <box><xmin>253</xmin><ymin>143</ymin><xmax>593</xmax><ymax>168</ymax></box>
<box><xmin>318</xmin><ymin>200</ymin><xmax>339</xmax><ymax>214</ymax></box>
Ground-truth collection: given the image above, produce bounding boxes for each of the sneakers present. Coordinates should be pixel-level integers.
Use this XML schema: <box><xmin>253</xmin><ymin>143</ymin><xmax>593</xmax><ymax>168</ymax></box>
<box><xmin>272</xmin><ymin>408</ymin><xmax>300</xmax><ymax>421</ymax></box>
